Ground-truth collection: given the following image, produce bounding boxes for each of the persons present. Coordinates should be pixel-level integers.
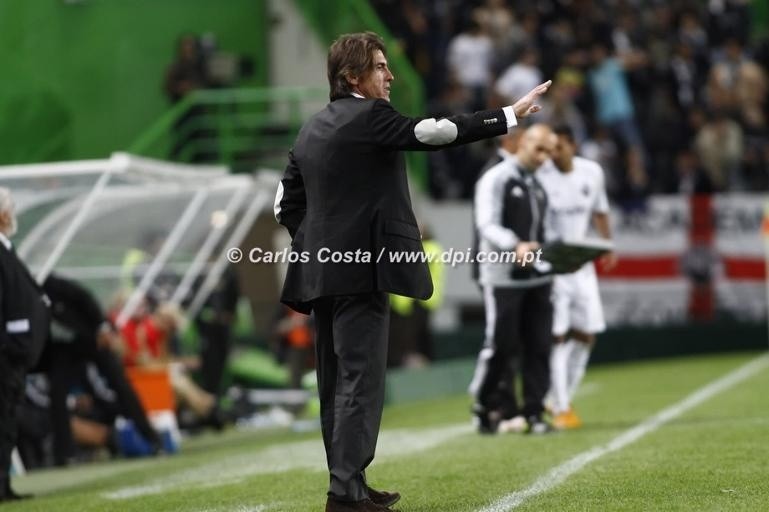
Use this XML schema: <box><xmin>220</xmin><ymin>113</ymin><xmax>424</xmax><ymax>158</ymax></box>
<box><xmin>19</xmin><ymin>227</ymin><xmax>314</xmax><ymax>469</ymax></box>
<box><xmin>368</xmin><ymin>0</ymin><xmax>769</xmax><ymax>204</ymax></box>
<box><xmin>472</xmin><ymin>124</ymin><xmax>562</xmax><ymax>435</ymax></box>
<box><xmin>538</xmin><ymin>126</ymin><xmax>618</xmax><ymax>430</ymax></box>
<box><xmin>271</xmin><ymin>29</ymin><xmax>553</xmax><ymax>512</ymax></box>
<box><xmin>194</xmin><ymin>32</ymin><xmax>258</xmax><ymax>175</ymax></box>
<box><xmin>163</xmin><ymin>30</ymin><xmax>209</xmax><ymax>168</ymax></box>
<box><xmin>466</xmin><ymin>125</ymin><xmax>533</xmax><ymax>292</ymax></box>
<box><xmin>2</xmin><ymin>186</ymin><xmax>46</xmax><ymax>500</ymax></box>
<box><xmin>393</xmin><ymin>231</ymin><xmax>441</xmax><ymax>369</ymax></box>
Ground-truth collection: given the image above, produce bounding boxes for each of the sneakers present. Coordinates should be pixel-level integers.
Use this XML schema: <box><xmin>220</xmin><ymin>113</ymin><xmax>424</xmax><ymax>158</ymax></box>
<box><xmin>480</xmin><ymin>410</ymin><xmax>547</xmax><ymax>433</ymax></box>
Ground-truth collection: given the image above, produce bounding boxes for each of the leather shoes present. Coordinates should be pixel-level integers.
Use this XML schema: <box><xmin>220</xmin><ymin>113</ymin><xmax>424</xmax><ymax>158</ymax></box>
<box><xmin>326</xmin><ymin>487</ymin><xmax>401</xmax><ymax>512</ymax></box>
